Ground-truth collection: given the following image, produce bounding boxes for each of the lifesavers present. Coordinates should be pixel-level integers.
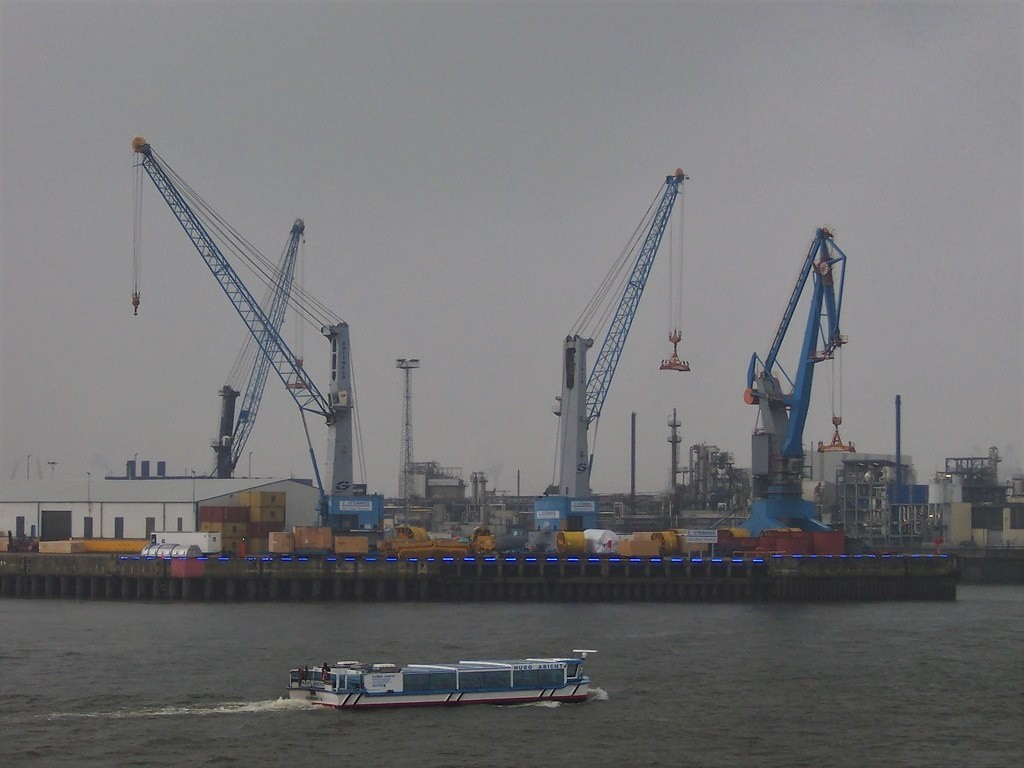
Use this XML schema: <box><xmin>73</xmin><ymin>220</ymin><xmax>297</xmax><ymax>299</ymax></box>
<box><xmin>297</xmin><ymin>667</ymin><xmax>304</xmax><ymax>678</ymax></box>
<box><xmin>322</xmin><ymin>666</ymin><xmax>328</xmax><ymax>673</ymax></box>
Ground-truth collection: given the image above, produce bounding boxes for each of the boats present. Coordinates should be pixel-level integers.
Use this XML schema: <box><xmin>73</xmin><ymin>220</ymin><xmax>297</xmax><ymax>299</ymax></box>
<box><xmin>288</xmin><ymin>650</ymin><xmax>598</xmax><ymax>710</ymax></box>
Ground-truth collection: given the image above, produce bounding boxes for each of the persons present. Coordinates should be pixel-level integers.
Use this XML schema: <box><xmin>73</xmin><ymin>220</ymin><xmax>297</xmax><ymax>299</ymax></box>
<box><xmin>297</xmin><ymin>668</ymin><xmax>304</xmax><ymax>686</ymax></box>
<box><xmin>322</xmin><ymin>662</ymin><xmax>330</xmax><ymax>680</ymax></box>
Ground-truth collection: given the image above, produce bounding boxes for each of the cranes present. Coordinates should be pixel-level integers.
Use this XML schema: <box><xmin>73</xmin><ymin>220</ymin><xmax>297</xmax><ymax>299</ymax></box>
<box><xmin>738</xmin><ymin>227</ymin><xmax>857</xmax><ymax>531</ymax></box>
<box><xmin>131</xmin><ymin>135</ymin><xmax>382</xmax><ymax>532</ymax></box>
<box><xmin>535</xmin><ymin>168</ymin><xmax>691</xmax><ymax>531</ymax></box>
